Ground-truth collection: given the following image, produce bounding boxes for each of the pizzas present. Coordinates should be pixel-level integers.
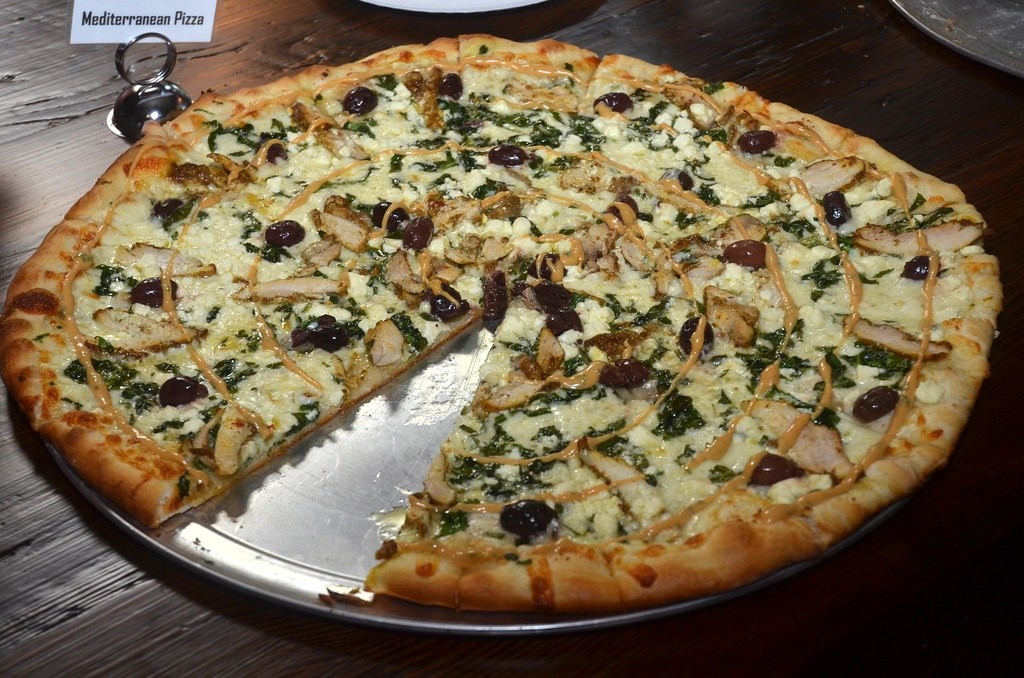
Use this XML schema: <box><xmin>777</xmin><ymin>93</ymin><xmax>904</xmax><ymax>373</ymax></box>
<box><xmin>0</xmin><ymin>35</ymin><xmax>998</xmax><ymax>611</ymax></box>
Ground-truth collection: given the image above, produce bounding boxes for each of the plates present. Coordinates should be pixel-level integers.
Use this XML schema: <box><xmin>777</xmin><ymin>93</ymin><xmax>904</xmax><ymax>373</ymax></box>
<box><xmin>888</xmin><ymin>0</ymin><xmax>1024</xmax><ymax>80</ymax></box>
<box><xmin>20</xmin><ymin>311</ymin><xmax>940</xmax><ymax>636</ymax></box>
<box><xmin>359</xmin><ymin>0</ymin><xmax>550</xmax><ymax>13</ymax></box>
